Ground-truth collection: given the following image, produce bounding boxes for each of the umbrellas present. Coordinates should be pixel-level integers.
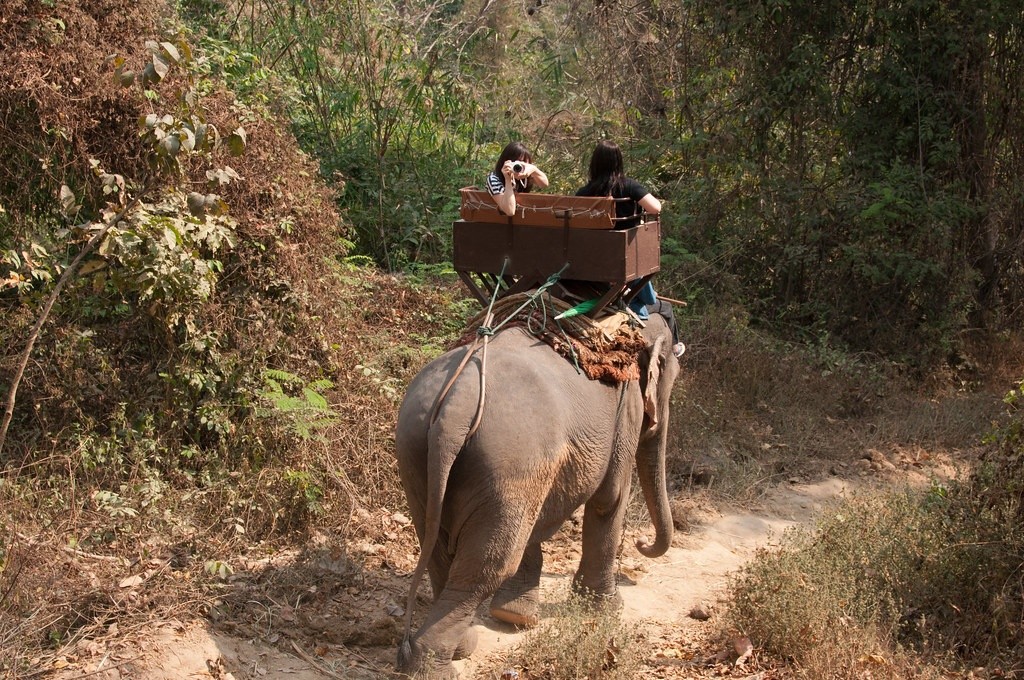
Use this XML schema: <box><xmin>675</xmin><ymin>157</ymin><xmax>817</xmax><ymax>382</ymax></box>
<box><xmin>554</xmin><ymin>297</ymin><xmax>600</xmax><ymax>321</ymax></box>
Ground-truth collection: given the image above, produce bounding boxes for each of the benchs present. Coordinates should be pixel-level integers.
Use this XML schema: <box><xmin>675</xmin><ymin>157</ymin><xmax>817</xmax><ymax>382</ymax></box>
<box><xmin>453</xmin><ymin>186</ymin><xmax>660</xmax><ymax>317</ymax></box>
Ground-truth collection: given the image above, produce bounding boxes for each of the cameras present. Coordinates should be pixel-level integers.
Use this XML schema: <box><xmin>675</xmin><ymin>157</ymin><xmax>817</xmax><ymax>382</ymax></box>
<box><xmin>509</xmin><ymin>162</ymin><xmax>525</xmax><ymax>173</ymax></box>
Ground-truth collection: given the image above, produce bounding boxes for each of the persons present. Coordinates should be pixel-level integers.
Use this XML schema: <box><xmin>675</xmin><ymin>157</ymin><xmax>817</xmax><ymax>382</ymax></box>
<box><xmin>574</xmin><ymin>139</ymin><xmax>661</xmax><ymax>230</ymax></box>
<box><xmin>624</xmin><ymin>277</ymin><xmax>685</xmax><ymax>357</ymax></box>
<box><xmin>484</xmin><ymin>141</ymin><xmax>549</xmax><ymax>216</ymax></box>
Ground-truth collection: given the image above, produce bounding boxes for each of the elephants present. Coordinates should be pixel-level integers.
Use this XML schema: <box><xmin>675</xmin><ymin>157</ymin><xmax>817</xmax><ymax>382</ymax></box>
<box><xmin>395</xmin><ymin>312</ymin><xmax>680</xmax><ymax>680</ymax></box>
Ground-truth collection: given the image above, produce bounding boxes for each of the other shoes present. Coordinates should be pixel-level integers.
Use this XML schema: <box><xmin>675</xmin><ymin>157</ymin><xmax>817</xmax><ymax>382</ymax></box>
<box><xmin>671</xmin><ymin>340</ymin><xmax>688</xmax><ymax>362</ymax></box>
